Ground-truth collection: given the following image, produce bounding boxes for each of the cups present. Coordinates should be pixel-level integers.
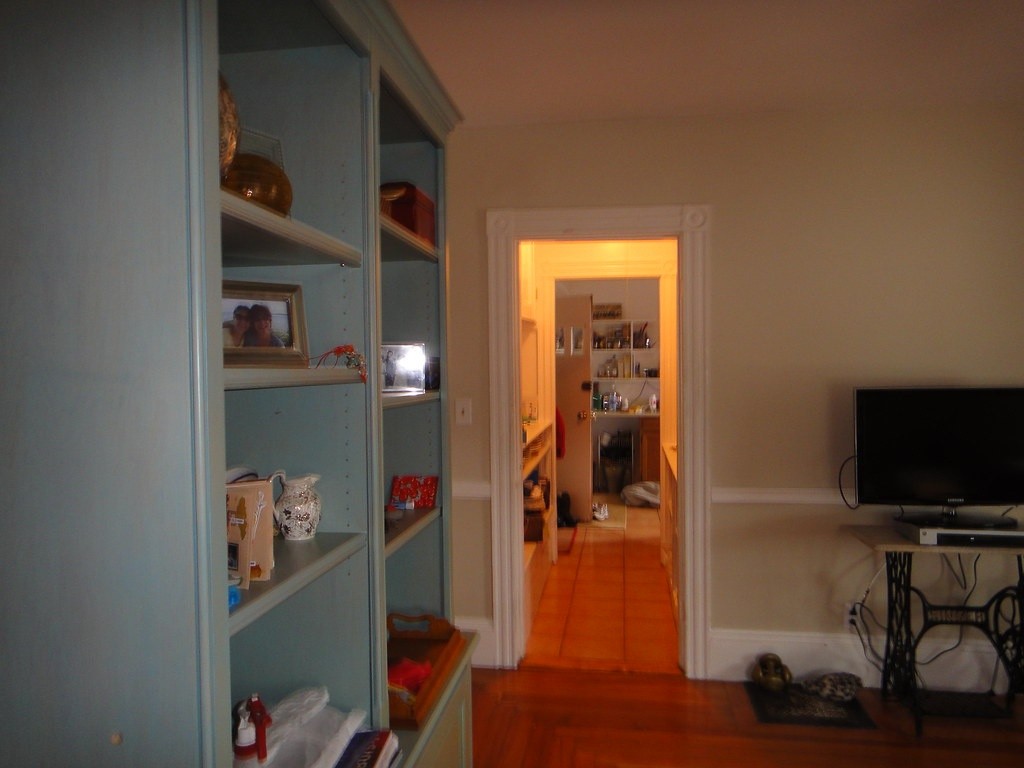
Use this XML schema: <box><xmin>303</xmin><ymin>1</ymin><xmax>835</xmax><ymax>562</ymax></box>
<box><xmin>648</xmin><ymin>368</ymin><xmax>657</xmax><ymax>377</ymax></box>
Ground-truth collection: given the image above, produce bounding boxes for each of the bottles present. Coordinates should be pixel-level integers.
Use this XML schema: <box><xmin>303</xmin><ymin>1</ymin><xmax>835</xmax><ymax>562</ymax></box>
<box><xmin>609</xmin><ymin>385</ymin><xmax>616</xmax><ymax>411</ymax></box>
<box><xmin>621</xmin><ymin>398</ymin><xmax>629</xmax><ymax>411</ymax></box>
<box><xmin>598</xmin><ymin>359</ymin><xmax>612</xmax><ymax>377</ymax></box>
<box><xmin>650</xmin><ymin>394</ymin><xmax>657</xmax><ymax>410</ymax></box>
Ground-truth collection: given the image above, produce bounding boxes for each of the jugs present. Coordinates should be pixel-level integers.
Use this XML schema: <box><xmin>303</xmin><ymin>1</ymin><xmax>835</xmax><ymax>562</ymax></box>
<box><xmin>267</xmin><ymin>468</ymin><xmax>322</xmax><ymax>542</ymax></box>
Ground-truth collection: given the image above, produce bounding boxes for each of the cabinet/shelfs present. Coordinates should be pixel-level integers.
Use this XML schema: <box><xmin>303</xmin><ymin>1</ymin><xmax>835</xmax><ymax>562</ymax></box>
<box><xmin>590</xmin><ymin>316</ymin><xmax>661</xmax><ymax>417</ymax></box>
<box><xmin>0</xmin><ymin>0</ymin><xmax>482</xmax><ymax>768</ymax></box>
<box><xmin>519</xmin><ymin>417</ymin><xmax>554</xmax><ymax>622</ymax></box>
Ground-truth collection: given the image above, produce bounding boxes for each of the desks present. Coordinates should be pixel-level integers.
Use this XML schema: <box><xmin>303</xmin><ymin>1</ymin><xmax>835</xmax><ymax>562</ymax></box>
<box><xmin>840</xmin><ymin>523</ymin><xmax>1024</xmax><ymax>738</ymax></box>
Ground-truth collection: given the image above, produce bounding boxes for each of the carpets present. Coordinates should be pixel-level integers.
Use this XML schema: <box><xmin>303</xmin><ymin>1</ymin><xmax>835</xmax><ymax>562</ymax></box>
<box><xmin>742</xmin><ymin>679</ymin><xmax>876</xmax><ymax>732</ymax></box>
<box><xmin>556</xmin><ymin>526</ymin><xmax>577</xmax><ymax>554</ymax></box>
<box><xmin>579</xmin><ymin>493</ymin><xmax>625</xmax><ymax>529</ymax></box>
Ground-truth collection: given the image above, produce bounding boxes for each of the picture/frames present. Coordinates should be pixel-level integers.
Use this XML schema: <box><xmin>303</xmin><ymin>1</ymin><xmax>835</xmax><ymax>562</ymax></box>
<box><xmin>380</xmin><ymin>341</ymin><xmax>427</xmax><ymax>394</ymax></box>
<box><xmin>220</xmin><ymin>279</ymin><xmax>312</xmax><ymax>370</ymax></box>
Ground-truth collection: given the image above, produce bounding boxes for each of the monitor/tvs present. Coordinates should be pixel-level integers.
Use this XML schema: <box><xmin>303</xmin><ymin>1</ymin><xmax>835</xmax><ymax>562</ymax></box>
<box><xmin>853</xmin><ymin>387</ymin><xmax>1024</xmax><ymax>528</ymax></box>
